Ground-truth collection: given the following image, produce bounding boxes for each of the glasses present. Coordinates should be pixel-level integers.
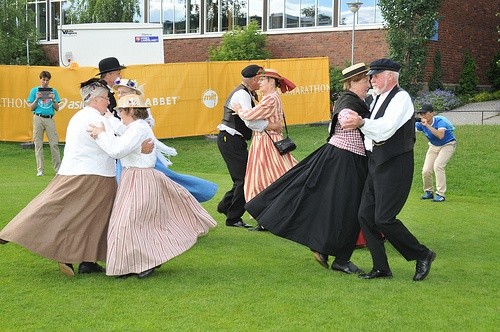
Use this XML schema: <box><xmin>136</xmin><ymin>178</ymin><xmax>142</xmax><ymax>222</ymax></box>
<box><xmin>118</xmin><ymin>90</ymin><xmax>133</xmax><ymax>94</ymax></box>
<box><xmin>99</xmin><ymin>96</ymin><xmax>110</xmax><ymax>102</ymax></box>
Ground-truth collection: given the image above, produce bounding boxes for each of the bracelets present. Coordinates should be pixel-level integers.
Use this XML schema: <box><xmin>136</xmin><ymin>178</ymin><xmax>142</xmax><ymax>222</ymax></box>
<box><xmin>53</xmin><ymin>100</ymin><xmax>56</xmax><ymax>103</ymax></box>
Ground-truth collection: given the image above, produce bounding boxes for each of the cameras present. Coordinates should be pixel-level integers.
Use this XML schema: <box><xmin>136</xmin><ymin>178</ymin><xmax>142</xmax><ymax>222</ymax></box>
<box><xmin>415</xmin><ymin>115</ymin><xmax>421</xmax><ymax>122</ymax></box>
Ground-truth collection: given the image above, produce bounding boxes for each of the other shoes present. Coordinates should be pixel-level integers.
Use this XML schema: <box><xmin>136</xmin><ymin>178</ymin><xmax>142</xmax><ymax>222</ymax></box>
<box><xmin>77</xmin><ymin>262</ymin><xmax>105</xmax><ymax>274</ymax></box>
<box><xmin>432</xmin><ymin>195</ymin><xmax>445</xmax><ymax>202</ymax></box>
<box><xmin>36</xmin><ymin>170</ymin><xmax>44</xmax><ymax>176</ymax></box>
<box><xmin>248</xmin><ymin>224</ymin><xmax>268</xmax><ymax>231</ymax></box>
<box><xmin>420</xmin><ymin>192</ymin><xmax>433</xmax><ymax>200</ymax></box>
<box><xmin>310</xmin><ymin>248</ymin><xmax>329</xmax><ymax>269</ymax></box>
<box><xmin>58</xmin><ymin>261</ymin><xmax>75</xmax><ymax>277</ymax></box>
<box><xmin>331</xmin><ymin>261</ymin><xmax>357</xmax><ymax>275</ymax></box>
<box><xmin>225</xmin><ymin>220</ymin><xmax>252</xmax><ymax>229</ymax></box>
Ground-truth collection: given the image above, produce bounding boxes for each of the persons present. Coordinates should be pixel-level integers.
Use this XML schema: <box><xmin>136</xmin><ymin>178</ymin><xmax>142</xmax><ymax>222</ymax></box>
<box><xmin>0</xmin><ymin>58</ymin><xmax>436</xmax><ymax>282</ymax></box>
<box><xmin>27</xmin><ymin>70</ymin><xmax>61</xmax><ymax>176</ymax></box>
<box><xmin>414</xmin><ymin>105</ymin><xmax>457</xmax><ymax>201</ymax></box>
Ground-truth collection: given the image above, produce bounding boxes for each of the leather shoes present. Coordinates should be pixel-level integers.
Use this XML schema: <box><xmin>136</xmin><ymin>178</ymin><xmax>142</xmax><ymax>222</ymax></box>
<box><xmin>358</xmin><ymin>269</ymin><xmax>394</xmax><ymax>279</ymax></box>
<box><xmin>413</xmin><ymin>249</ymin><xmax>436</xmax><ymax>281</ymax></box>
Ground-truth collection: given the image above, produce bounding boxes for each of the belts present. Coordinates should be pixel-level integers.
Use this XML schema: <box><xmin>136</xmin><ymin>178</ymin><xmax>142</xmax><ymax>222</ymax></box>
<box><xmin>34</xmin><ymin>112</ymin><xmax>53</xmax><ymax>118</ymax></box>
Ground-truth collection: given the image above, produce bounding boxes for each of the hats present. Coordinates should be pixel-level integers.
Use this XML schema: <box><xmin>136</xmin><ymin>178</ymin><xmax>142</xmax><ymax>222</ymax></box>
<box><xmin>415</xmin><ymin>104</ymin><xmax>433</xmax><ymax>114</ymax></box>
<box><xmin>338</xmin><ymin>63</ymin><xmax>371</xmax><ymax>81</ymax></box>
<box><xmin>365</xmin><ymin>58</ymin><xmax>400</xmax><ymax>75</ymax></box>
<box><xmin>112</xmin><ymin>79</ymin><xmax>142</xmax><ymax>95</ymax></box>
<box><xmin>113</xmin><ymin>94</ymin><xmax>151</xmax><ymax>109</ymax></box>
<box><xmin>256</xmin><ymin>69</ymin><xmax>280</xmax><ymax>79</ymax></box>
<box><xmin>240</xmin><ymin>64</ymin><xmax>264</xmax><ymax>78</ymax></box>
<box><xmin>95</xmin><ymin>57</ymin><xmax>128</xmax><ymax>76</ymax></box>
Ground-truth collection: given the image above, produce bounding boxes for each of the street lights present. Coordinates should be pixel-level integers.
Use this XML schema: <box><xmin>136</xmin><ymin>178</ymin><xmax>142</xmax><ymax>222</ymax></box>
<box><xmin>346</xmin><ymin>2</ymin><xmax>364</xmax><ymax>66</ymax></box>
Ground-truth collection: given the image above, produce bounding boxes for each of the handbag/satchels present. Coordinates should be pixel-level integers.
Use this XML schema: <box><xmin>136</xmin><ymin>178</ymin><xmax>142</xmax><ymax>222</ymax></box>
<box><xmin>272</xmin><ymin>138</ymin><xmax>297</xmax><ymax>156</ymax></box>
